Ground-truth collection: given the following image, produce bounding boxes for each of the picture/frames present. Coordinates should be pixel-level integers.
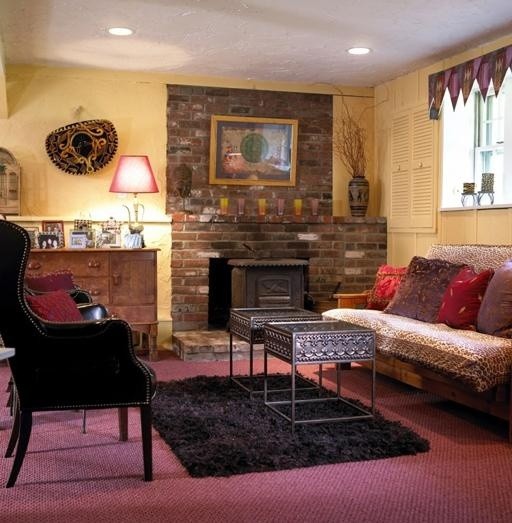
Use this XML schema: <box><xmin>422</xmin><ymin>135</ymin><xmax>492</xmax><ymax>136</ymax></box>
<box><xmin>36</xmin><ymin>232</ymin><xmax>63</xmax><ymax>249</ymax></box>
<box><xmin>209</xmin><ymin>115</ymin><xmax>297</xmax><ymax>187</ymax></box>
<box><xmin>42</xmin><ymin>220</ymin><xmax>65</xmax><ymax>247</ymax></box>
<box><xmin>70</xmin><ymin>235</ymin><xmax>86</xmax><ymax>249</ymax></box>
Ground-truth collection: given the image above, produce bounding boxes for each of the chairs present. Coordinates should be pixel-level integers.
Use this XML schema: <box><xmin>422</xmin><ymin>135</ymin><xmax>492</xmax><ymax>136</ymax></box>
<box><xmin>6</xmin><ymin>291</ymin><xmax>108</xmax><ymax>416</ymax></box>
<box><xmin>0</xmin><ymin>219</ymin><xmax>159</xmax><ymax>488</ymax></box>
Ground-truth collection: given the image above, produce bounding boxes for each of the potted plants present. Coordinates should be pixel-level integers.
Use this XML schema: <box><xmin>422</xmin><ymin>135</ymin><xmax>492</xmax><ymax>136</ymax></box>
<box><xmin>310</xmin><ymin>81</ymin><xmax>390</xmax><ymax>217</ymax></box>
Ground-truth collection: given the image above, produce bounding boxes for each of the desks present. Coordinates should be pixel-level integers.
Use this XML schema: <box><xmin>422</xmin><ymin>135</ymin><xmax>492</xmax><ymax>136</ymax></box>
<box><xmin>26</xmin><ymin>249</ymin><xmax>162</xmax><ymax>362</ymax></box>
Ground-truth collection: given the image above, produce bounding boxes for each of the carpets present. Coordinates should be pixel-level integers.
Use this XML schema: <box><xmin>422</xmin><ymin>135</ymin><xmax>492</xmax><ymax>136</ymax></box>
<box><xmin>150</xmin><ymin>371</ymin><xmax>432</xmax><ymax>478</ymax></box>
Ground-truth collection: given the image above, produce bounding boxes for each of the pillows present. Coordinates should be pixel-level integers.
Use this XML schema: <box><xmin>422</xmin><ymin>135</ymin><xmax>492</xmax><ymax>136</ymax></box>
<box><xmin>26</xmin><ymin>289</ymin><xmax>83</xmax><ymax>322</ymax></box>
<box><xmin>478</xmin><ymin>261</ymin><xmax>512</xmax><ymax>333</ymax></box>
<box><xmin>384</xmin><ymin>257</ymin><xmax>465</xmax><ymax>322</ymax></box>
<box><xmin>438</xmin><ymin>265</ymin><xmax>494</xmax><ymax>330</ymax></box>
<box><xmin>26</xmin><ymin>271</ymin><xmax>73</xmax><ymax>291</ymax></box>
<box><xmin>367</xmin><ymin>265</ymin><xmax>406</xmax><ymax>311</ymax></box>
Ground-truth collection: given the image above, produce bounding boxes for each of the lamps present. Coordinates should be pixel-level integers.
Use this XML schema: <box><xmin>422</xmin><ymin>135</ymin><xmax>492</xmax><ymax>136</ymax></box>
<box><xmin>109</xmin><ymin>155</ymin><xmax>160</xmax><ymax>247</ymax></box>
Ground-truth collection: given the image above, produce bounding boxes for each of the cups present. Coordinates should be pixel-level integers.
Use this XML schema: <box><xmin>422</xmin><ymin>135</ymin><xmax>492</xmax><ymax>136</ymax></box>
<box><xmin>294</xmin><ymin>199</ymin><xmax>301</xmax><ymax>215</ymax></box>
<box><xmin>237</xmin><ymin>198</ymin><xmax>246</xmax><ymax>215</ymax></box>
<box><xmin>258</xmin><ymin>199</ymin><xmax>267</xmax><ymax>216</ymax></box>
<box><xmin>220</xmin><ymin>198</ymin><xmax>228</xmax><ymax>215</ymax></box>
<box><xmin>277</xmin><ymin>199</ymin><xmax>285</xmax><ymax>216</ymax></box>
<box><xmin>311</xmin><ymin>199</ymin><xmax>318</xmax><ymax>217</ymax></box>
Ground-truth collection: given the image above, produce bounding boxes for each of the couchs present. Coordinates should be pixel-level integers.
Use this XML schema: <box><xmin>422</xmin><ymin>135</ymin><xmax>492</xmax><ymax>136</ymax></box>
<box><xmin>321</xmin><ymin>244</ymin><xmax>512</xmax><ymax>422</ymax></box>
